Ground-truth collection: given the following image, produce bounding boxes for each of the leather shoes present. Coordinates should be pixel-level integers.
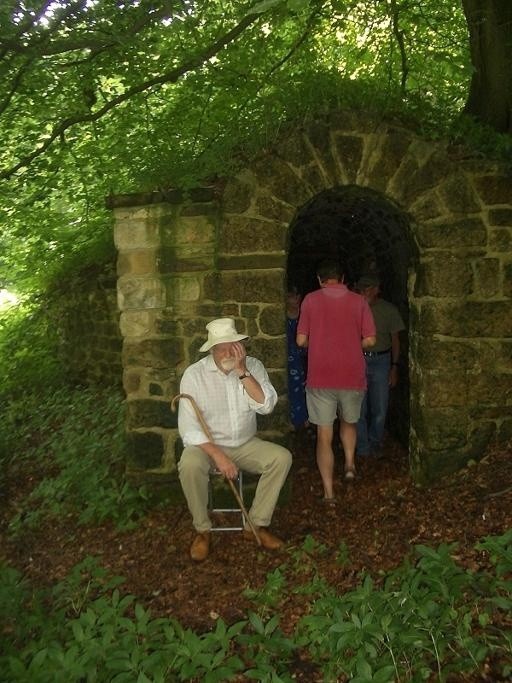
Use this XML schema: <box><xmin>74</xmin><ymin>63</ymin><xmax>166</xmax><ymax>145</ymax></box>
<box><xmin>242</xmin><ymin>529</ymin><xmax>284</xmax><ymax>550</ymax></box>
<box><xmin>191</xmin><ymin>532</ymin><xmax>211</xmax><ymax>561</ymax></box>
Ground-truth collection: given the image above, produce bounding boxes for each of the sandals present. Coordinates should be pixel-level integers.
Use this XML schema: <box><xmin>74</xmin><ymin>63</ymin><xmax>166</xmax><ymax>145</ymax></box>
<box><xmin>342</xmin><ymin>466</ymin><xmax>356</xmax><ymax>483</ymax></box>
<box><xmin>323</xmin><ymin>498</ymin><xmax>338</xmax><ymax>512</ymax></box>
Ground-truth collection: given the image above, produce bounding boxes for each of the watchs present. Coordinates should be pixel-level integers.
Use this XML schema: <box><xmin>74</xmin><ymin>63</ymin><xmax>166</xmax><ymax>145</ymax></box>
<box><xmin>238</xmin><ymin>370</ymin><xmax>251</xmax><ymax>379</ymax></box>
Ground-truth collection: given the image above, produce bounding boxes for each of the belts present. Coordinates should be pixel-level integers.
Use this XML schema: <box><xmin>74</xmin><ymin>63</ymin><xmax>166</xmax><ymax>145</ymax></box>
<box><xmin>363</xmin><ymin>347</ymin><xmax>392</xmax><ymax>357</ymax></box>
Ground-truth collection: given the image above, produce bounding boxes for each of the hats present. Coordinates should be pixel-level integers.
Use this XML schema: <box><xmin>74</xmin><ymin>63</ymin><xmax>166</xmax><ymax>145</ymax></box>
<box><xmin>199</xmin><ymin>318</ymin><xmax>249</xmax><ymax>353</ymax></box>
<box><xmin>358</xmin><ymin>275</ymin><xmax>379</xmax><ymax>286</ymax></box>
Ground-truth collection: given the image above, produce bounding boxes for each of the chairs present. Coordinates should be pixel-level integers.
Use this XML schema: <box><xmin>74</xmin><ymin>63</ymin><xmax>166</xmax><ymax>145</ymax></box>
<box><xmin>172</xmin><ymin>440</ymin><xmax>252</xmax><ymax>539</ymax></box>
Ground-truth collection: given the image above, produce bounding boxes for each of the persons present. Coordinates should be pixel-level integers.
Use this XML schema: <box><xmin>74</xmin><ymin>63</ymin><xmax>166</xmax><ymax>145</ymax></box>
<box><xmin>295</xmin><ymin>262</ymin><xmax>377</xmax><ymax>502</ymax></box>
<box><xmin>177</xmin><ymin>318</ymin><xmax>293</xmax><ymax>561</ymax></box>
<box><xmin>285</xmin><ymin>284</ymin><xmax>313</xmax><ymax>435</ymax></box>
<box><xmin>353</xmin><ymin>271</ymin><xmax>407</xmax><ymax>464</ymax></box>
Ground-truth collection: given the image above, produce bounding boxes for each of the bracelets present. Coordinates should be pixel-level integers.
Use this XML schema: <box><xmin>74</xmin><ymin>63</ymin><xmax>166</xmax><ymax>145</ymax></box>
<box><xmin>390</xmin><ymin>360</ymin><xmax>398</xmax><ymax>367</ymax></box>
